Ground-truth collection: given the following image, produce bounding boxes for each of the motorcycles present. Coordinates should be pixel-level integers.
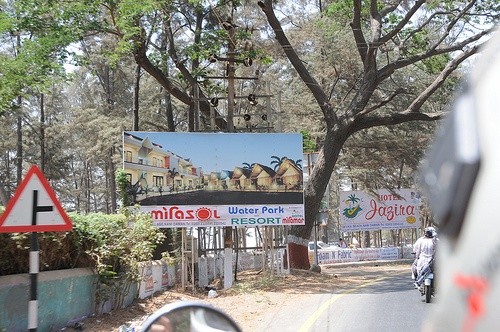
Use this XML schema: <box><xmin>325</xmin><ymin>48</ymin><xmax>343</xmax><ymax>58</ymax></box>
<box><xmin>410</xmin><ymin>252</ymin><xmax>434</xmax><ymax>303</ymax></box>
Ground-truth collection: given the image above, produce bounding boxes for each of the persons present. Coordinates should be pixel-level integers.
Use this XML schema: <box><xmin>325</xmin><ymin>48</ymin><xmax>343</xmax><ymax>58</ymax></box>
<box><xmin>411</xmin><ymin>227</ymin><xmax>440</xmax><ymax>290</ymax></box>
<box><xmin>339</xmin><ymin>237</ymin><xmax>348</xmax><ymax>248</ymax></box>
<box><xmin>415</xmin><ymin>227</ymin><xmax>436</xmax><ymax>275</ymax></box>
